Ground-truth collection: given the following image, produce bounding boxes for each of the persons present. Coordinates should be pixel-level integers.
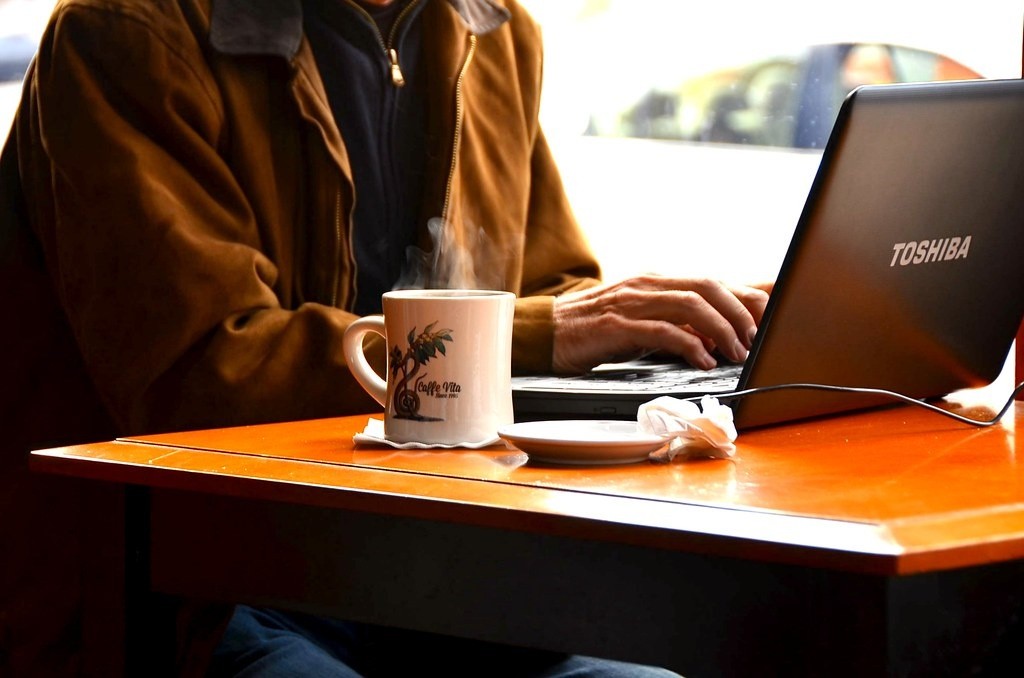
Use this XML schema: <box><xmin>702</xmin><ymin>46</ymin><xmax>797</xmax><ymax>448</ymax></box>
<box><xmin>0</xmin><ymin>0</ymin><xmax>776</xmax><ymax>678</ymax></box>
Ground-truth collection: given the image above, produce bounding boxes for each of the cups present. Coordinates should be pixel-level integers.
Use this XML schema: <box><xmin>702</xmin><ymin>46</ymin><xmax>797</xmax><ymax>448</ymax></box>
<box><xmin>342</xmin><ymin>288</ymin><xmax>516</xmax><ymax>444</ymax></box>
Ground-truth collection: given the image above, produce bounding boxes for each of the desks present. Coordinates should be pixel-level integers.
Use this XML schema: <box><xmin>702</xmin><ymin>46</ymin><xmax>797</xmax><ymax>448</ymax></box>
<box><xmin>30</xmin><ymin>351</ymin><xmax>1024</xmax><ymax>678</ymax></box>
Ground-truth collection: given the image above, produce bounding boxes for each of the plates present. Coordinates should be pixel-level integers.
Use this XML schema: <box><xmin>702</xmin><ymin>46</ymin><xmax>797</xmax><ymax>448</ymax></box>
<box><xmin>496</xmin><ymin>420</ymin><xmax>675</xmax><ymax>464</ymax></box>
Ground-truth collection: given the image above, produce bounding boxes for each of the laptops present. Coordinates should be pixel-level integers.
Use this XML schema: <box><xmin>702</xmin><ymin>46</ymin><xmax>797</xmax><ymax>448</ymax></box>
<box><xmin>501</xmin><ymin>79</ymin><xmax>1024</xmax><ymax>430</ymax></box>
